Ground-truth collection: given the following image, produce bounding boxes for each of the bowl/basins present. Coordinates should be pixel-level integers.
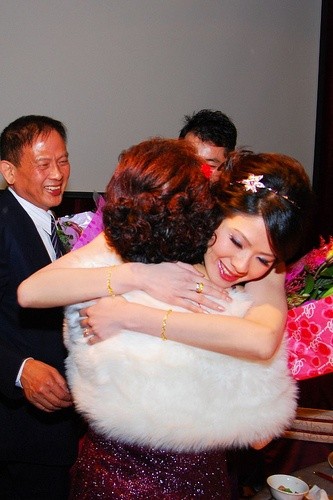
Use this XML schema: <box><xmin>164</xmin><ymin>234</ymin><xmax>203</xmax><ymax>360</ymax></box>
<box><xmin>267</xmin><ymin>474</ymin><xmax>309</xmax><ymax>500</ymax></box>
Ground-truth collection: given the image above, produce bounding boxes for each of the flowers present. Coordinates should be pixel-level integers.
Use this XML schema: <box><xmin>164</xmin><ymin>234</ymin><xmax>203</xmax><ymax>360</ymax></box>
<box><xmin>57</xmin><ymin>220</ymin><xmax>82</xmax><ymax>249</ymax></box>
<box><xmin>285</xmin><ymin>236</ymin><xmax>333</xmax><ymax>311</ymax></box>
<box><xmin>201</xmin><ymin>164</ymin><xmax>212</xmax><ymax>179</ymax></box>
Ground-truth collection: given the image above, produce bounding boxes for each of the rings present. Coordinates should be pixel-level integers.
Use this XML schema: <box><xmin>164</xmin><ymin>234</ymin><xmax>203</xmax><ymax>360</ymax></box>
<box><xmin>196</xmin><ymin>282</ymin><xmax>204</xmax><ymax>293</ymax></box>
<box><xmin>84</xmin><ymin>328</ymin><xmax>89</xmax><ymax>335</ymax></box>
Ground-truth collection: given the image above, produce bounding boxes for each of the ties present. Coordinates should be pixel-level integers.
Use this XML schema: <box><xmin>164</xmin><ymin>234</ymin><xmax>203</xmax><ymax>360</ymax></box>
<box><xmin>49</xmin><ymin>216</ymin><xmax>66</xmax><ymax>259</ymax></box>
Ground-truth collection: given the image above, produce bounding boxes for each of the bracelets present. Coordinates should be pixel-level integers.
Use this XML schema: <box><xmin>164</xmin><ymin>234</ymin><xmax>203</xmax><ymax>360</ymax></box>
<box><xmin>107</xmin><ymin>265</ymin><xmax>117</xmax><ymax>297</ymax></box>
<box><xmin>161</xmin><ymin>309</ymin><xmax>173</xmax><ymax>341</ymax></box>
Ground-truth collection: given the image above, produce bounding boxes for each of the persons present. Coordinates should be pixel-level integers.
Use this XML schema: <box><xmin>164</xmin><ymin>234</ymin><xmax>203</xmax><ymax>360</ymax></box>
<box><xmin>0</xmin><ymin>115</ymin><xmax>85</xmax><ymax>500</ymax></box>
<box><xmin>62</xmin><ymin>138</ymin><xmax>301</xmax><ymax>500</ymax></box>
<box><xmin>178</xmin><ymin>107</ymin><xmax>265</xmax><ymax>492</ymax></box>
<box><xmin>16</xmin><ymin>146</ymin><xmax>320</xmax><ymax>361</ymax></box>
